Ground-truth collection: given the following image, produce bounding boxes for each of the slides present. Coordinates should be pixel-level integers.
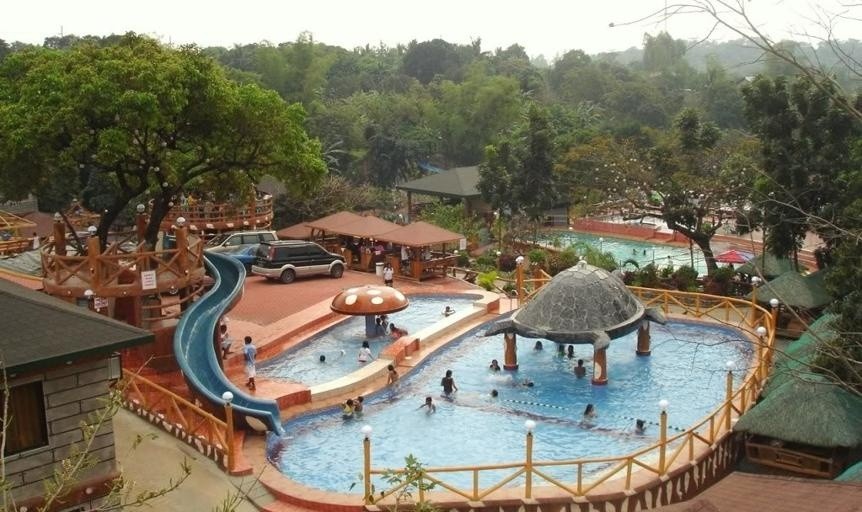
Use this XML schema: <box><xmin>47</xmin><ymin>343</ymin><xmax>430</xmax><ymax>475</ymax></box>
<box><xmin>162</xmin><ymin>231</ymin><xmax>285</xmax><ymax>435</ymax></box>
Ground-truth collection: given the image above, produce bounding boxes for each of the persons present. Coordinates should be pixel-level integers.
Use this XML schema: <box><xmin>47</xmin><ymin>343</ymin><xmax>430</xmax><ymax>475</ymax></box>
<box><xmin>489</xmin><ymin>341</ymin><xmax>587</xmax><ymax>397</ymax></box>
<box><xmin>634</xmin><ymin>418</ymin><xmax>645</xmax><ymax>437</ymax></box>
<box><xmin>30</xmin><ymin>232</ymin><xmax>40</xmax><ymax>251</ymax></box>
<box><xmin>354</xmin><ymin>395</ymin><xmax>366</xmax><ymax>418</ymax></box>
<box><xmin>400</xmin><ymin>244</ymin><xmax>431</xmax><ymax>277</ymax></box>
<box><xmin>376</xmin><ymin>312</ymin><xmax>408</xmax><ymax>341</ymax></box>
<box><xmin>220</xmin><ymin>324</ymin><xmax>234</xmax><ymax>360</ymax></box>
<box><xmin>316</xmin><ymin>350</ymin><xmax>343</xmax><ymax>365</ymax></box>
<box><xmin>444</xmin><ymin>306</ymin><xmax>456</xmax><ymax>317</ymax></box>
<box><xmin>340</xmin><ymin>399</ymin><xmax>356</xmax><ymax>420</ymax></box>
<box><xmin>727</xmin><ymin>262</ymin><xmax>761</xmax><ymax>285</ymax></box>
<box><xmin>632</xmin><ymin>246</ymin><xmax>646</xmax><ymax>257</ymax></box>
<box><xmin>386</xmin><ymin>364</ymin><xmax>401</xmax><ymax>393</ymax></box>
<box><xmin>440</xmin><ymin>370</ymin><xmax>459</xmax><ymax>394</ymax></box>
<box><xmin>243</xmin><ymin>335</ymin><xmax>257</xmax><ymax>392</ymax></box>
<box><xmin>341</xmin><ymin>238</ymin><xmax>385</xmax><ymax>268</ymax></box>
<box><xmin>86</xmin><ymin>220</ymin><xmax>98</xmax><ymax>233</ymax></box>
<box><xmin>358</xmin><ymin>340</ymin><xmax>378</xmax><ymax>366</ymax></box>
<box><xmin>583</xmin><ymin>403</ymin><xmax>596</xmax><ymax>418</ymax></box>
<box><xmin>668</xmin><ymin>256</ymin><xmax>673</xmax><ymax>266</ymax></box>
<box><xmin>415</xmin><ymin>396</ymin><xmax>436</xmax><ymax>417</ymax></box>
<box><xmin>382</xmin><ymin>262</ymin><xmax>394</xmax><ymax>289</ymax></box>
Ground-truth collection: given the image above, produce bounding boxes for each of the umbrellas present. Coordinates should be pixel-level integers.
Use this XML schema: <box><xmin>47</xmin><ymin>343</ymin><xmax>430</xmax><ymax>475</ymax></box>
<box><xmin>713</xmin><ymin>250</ymin><xmax>748</xmax><ymax>265</ymax></box>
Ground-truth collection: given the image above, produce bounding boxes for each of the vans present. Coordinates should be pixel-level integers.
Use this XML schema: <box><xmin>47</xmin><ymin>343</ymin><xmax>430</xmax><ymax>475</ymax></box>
<box><xmin>203</xmin><ymin>230</ymin><xmax>280</xmax><ymax>254</ymax></box>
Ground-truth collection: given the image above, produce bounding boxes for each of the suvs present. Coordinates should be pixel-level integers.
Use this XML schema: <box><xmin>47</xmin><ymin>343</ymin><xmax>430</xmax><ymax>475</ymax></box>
<box><xmin>251</xmin><ymin>239</ymin><xmax>347</xmax><ymax>284</ymax></box>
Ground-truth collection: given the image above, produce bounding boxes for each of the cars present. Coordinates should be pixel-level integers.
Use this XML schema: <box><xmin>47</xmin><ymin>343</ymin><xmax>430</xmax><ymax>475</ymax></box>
<box><xmin>218</xmin><ymin>243</ymin><xmax>261</xmax><ymax>276</ymax></box>
